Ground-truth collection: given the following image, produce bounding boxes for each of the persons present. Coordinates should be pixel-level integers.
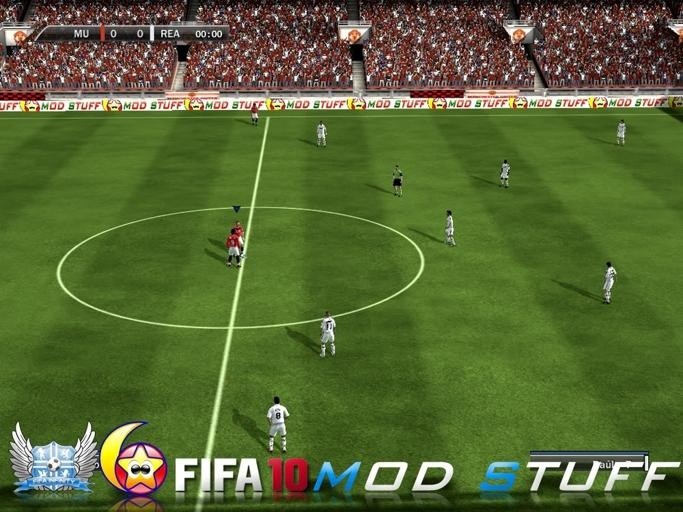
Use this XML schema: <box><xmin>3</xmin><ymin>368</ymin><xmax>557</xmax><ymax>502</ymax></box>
<box><xmin>316</xmin><ymin>120</ymin><xmax>328</xmax><ymax>148</ymax></box>
<box><xmin>318</xmin><ymin>311</ymin><xmax>336</xmax><ymax>359</ymax></box>
<box><xmin>226</xmin><ymin>229</ymin><xmax>242</xmax><ymax>267</ymax></box>
<box><xmin>602</xmin><ymin>262</ymin><xmax>617</xmax><ymax>304</ymax></box>
<box><xmin>266</xmin><ymin>396</ymin><xmax>290</xmax><ymax>453</ymax></box>
<box><xmin>0</xmin><ymin>1</ymin><xmax>683</xmax><ymax>91</ymax></box>
<box><xmin>500</xmin><ymin>160</ymin><xmax>511</xmax><ymax>188</ymax></box>
<box><xmin>250</xmin><ymin>103</ymin><xmax>258</xmax><ymax>125</ymax></box>
<box><xmin>392</xmin><ymin>165</ymin><xmax>403</xmax><ymax>198</ymax></box>
<box><xmin>234</xmin><ymin>220</ymin><xmax>244</xmax><ymax>258</ymax></box>
<box><xmin>616</xmin><ymin>119</ymin><xmax>627</xmax><ymax>145</ymax></box>
<box><xmin>444</xmin><ymin>209</ymin><xmax>456</xmax><ymax>247</ymax></box>
